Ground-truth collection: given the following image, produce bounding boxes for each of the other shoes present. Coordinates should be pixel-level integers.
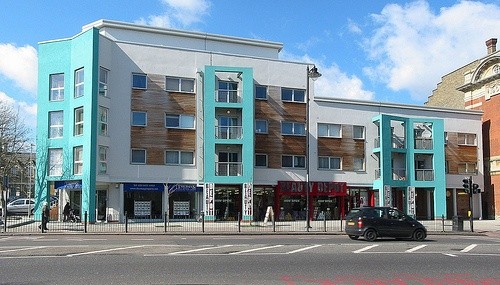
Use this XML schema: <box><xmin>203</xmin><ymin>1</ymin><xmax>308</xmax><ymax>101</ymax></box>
<box><xmin>45</xmin><ymin>228</ymin><xmax>49</xmax><ymax>230</ymax></box>
<box><xmin>38</xmin><ymin>226</ymin><xmax>41</xmax><ymax>229</ymax></box>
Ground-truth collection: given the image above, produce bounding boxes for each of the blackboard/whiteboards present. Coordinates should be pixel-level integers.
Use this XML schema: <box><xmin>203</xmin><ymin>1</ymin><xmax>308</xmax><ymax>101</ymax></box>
<box><xmin>264</xmin><ymin>206</ymin><xmax>274</xmax><ymax>223</ymax></box>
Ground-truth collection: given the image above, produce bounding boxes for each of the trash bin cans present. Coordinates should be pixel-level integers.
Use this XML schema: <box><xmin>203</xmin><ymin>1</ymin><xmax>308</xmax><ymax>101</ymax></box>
<box><xmin>452</xmin><ymin>216</ymin><xmax>464</xmax><ymax>231</ymax></box>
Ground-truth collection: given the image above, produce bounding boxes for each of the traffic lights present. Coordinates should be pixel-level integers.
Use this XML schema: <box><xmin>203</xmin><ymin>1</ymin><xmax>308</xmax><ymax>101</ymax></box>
<box><xmin>462</xmin><ymin>178</ymin><xmax>471</xmax><ymax>193</ymax></box>
<box><xmin>473</xmin><ymin>183</ymin><xmax>479</xmax><ymax>194</ymax></box>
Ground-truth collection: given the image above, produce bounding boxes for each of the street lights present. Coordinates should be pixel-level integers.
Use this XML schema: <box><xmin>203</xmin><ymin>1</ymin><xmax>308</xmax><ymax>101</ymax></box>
<box><xmin>28</xmin><ymin>143</ymin><xmax>36</xmax><ymax>219</ymax></box>
<box><xmin>305</xmin><ymin>63</ymin><xmax>323</xmax><ymax>229</ymax></box>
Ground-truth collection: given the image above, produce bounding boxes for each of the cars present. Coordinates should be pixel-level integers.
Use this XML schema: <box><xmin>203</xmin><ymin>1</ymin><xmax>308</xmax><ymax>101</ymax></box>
<box><xmin>343</xmin><ymin>205</ymin><xmax>428</xmax><ymax>242</ymax></box>
<box><xmin>0</xmin><ymin>197</ymin><xmax>36</xmax><ymax>217</ymax></box>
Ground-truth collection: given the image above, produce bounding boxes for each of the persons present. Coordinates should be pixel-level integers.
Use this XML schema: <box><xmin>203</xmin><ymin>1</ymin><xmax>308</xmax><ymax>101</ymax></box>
<box><xmin>38</xmin><ymin>202</ymin><xmax>50</xmax><ymax>230</ymax></box>
<box><xmin>61</xmin><ymin>201</ymin><xmax>72</xmax><ymax>222</ymax></box>
